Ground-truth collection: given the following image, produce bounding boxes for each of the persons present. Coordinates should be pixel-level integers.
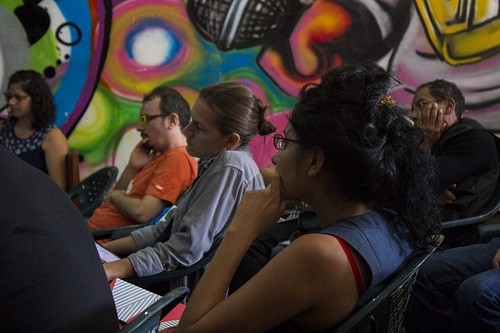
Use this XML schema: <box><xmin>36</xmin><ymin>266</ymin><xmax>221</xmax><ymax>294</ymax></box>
<box><xmin>103</xmin><ymin>81</ymin><xmax>267</xmax><ymax>297</ymax></box>
<box><xmin>0</xmin><ymin>147</ymin><xmax>119</xmax><ymax>333</ymax></box>
<box><xmin>411</xmin><ymin>79</ymin><xmax>500</xmax><ymax>222</ymax></box>
<box><xmin>157</xmin><ymin>62</ymin><xmax>434</xmax><ymax>333</ymax></box>
<box><xmin>406</xmin><ymin>239</ymin><xmax>500</xmax><ymax>333</ymax></box>
<box><xmin>0</xmin><ymin>70</ymin><xmax>67</xmax><ymax>193</ymax></box>
<box><xmin>86</xmin><ymin>85</ymin><xmax>199</xmax><ymax>245</ymax></box>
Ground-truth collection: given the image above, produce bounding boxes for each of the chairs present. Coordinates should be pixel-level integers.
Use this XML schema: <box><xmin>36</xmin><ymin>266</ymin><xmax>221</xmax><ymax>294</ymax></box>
<box><xmin>121</xmin><ymin>287</ymin><xmax>193</xmax><ymax>333</ymax></box>
<box><xmin>119</xmin><ymin>237</ymin><xmax>222</xmax><ymax>303</ymax></box>
<box><xmin>438</xmin><ymin>197</ymin><xmax>500</xmax><ymax>232</ymax></box>
<box><xmin>334</xmin><ymin>235</ymin><xmax>445</xmax><ymax>333</ymax></box>
<box><xmin>90</xmin><ymin>221</ymin><xmax>158</xmax><ymax>241</ymax></box>
<box><xmin>65</xmin><ymin>149</ymin><xmax>81</xmax><ymax>208</ymax></box>
<box><xmin>67</xmin><ymin>166</ymin><xmax>119</xmax><ymax>217</ymax></box>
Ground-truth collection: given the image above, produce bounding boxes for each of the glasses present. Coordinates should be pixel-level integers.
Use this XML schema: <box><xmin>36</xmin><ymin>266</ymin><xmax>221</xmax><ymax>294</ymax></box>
<box><xmin>4</xmin><ymin>91</ymin><xmax>29</xmax><ymax>102</ymax></box>
<box><xmin>407</xmin><ymin>99</ymin><xmax>439</xmax><ymax>112</ymax></box>
<box><xmin>139</xmin><ymin>113</ymin><xmax>167</xmax><ymax>124</ymax></box>
<box><xmin>273</xmin><ymin>134</ymin><xmax>312</xmax><ymax>150</ymax></box>
